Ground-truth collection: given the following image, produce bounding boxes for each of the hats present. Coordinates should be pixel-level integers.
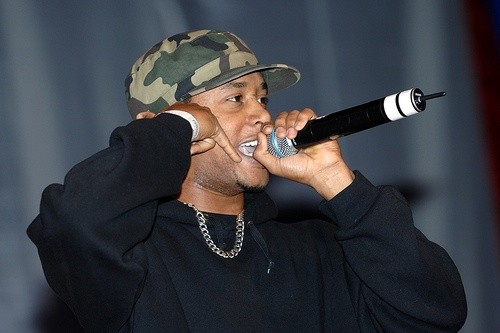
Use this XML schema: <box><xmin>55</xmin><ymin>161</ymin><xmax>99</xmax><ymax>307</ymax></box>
<box><xmin>125</xmin><ymin>28</ymin><xmax>301</xmax><ymax>121</ymax></box>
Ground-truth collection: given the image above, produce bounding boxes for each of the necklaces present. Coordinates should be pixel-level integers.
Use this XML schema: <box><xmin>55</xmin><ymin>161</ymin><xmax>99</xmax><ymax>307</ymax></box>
<box><xmin>181</xmin><ymin>202</ymin><xmax>246</xmax><ymax>258</ymax></box>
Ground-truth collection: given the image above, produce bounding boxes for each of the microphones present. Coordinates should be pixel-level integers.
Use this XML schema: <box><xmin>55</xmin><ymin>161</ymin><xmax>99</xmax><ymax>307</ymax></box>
<box><xmin>266</xmin><ymin>88</ymin><xmax>446</xmax><ymax>160</ymax></box>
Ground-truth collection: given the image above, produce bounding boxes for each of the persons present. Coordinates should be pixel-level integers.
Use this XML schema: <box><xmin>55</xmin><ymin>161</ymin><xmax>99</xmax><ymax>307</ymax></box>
<box><xmin>27</xmin><ymin>29</ymin><xmax>467</xmax><ymax>333</ymax></box>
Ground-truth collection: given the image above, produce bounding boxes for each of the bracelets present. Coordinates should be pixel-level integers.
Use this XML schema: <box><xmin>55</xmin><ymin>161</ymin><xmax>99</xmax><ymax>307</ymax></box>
<box><xmin>153</xmin><ymin>109</ymin><xmax>199</xmax><ymax>142</ymax></box>
